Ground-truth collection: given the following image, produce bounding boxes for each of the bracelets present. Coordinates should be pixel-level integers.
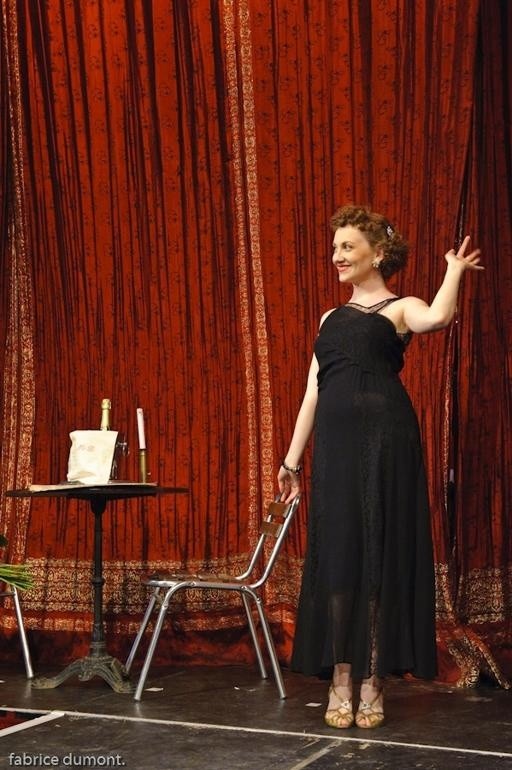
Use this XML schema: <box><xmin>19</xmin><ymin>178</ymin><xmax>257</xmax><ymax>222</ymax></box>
<box><xmin>280</xmin><ymin>461</ymin><xmax>303</xmax><ymax>475</ymax></box>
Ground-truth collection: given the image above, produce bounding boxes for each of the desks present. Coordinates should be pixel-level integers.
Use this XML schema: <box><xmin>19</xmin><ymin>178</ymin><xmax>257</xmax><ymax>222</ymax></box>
<box><xmin>3</xmin><ymin>482</ymin><xmax>191</xmax><ymax>696</ymax></box>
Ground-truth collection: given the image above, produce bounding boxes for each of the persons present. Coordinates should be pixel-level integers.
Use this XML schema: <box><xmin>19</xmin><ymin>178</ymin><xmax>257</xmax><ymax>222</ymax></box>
<box><xmin>274</xmin><ymin>205</ymin><xmax>487</xmax><ymax>728</ymax></box>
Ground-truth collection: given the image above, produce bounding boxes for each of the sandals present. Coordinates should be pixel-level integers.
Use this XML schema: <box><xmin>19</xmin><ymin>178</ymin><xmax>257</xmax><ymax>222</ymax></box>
<box><xmin>354</xmin><ymin>678</ymin><xmax>385</xmax><ymax>729</ymax></box>
<box><xmin>324</xmin><ymin>671</ymin><xmax>355</xmax><ymax>728</ymax></box>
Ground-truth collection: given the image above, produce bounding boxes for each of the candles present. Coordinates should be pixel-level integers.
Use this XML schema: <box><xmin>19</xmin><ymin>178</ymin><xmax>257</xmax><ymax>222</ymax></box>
<box><xmin>134</xmin><ymin>408</ymin><xmax>146</xmax><ymax>450</ymax></box>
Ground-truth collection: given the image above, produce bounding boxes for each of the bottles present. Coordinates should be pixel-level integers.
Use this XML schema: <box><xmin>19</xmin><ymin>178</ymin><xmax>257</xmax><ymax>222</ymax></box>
<box><xmin>100</xmin><ymin>399</ymin><xmax>118</xmax><ymax>480</ymax></box>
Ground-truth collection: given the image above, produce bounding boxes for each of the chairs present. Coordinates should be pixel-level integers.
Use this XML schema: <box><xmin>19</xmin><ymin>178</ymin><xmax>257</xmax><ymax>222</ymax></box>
<box><xmin>121</xmin><ymin>490</ymin><xmax>305</xmax><ymax>705</ymax></box>
<box><xmin>0</xmin><ymin>535</ymin><xmax>38</xmax><ymax>680</ymax></box>
